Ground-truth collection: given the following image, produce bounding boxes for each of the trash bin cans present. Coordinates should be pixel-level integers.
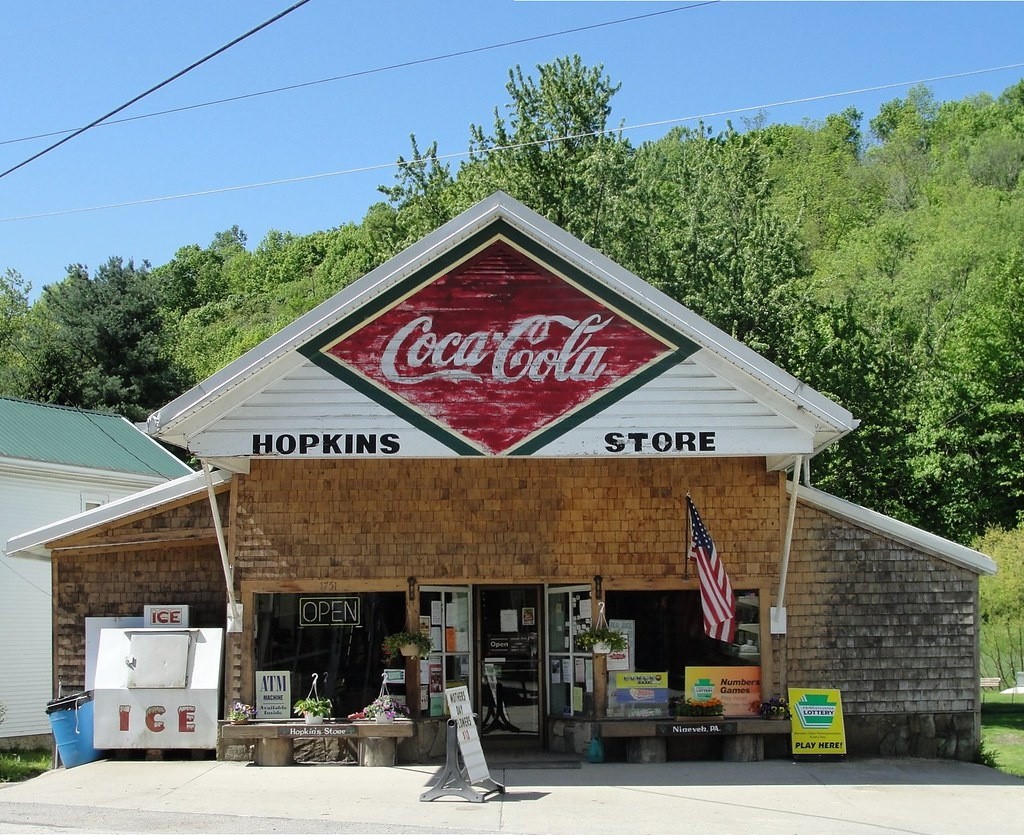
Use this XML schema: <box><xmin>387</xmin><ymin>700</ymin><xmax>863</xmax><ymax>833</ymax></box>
<box><xmin>45</xmin><ymin>690</ymin><xmax>103</xmax><ymax>769</ymax></box>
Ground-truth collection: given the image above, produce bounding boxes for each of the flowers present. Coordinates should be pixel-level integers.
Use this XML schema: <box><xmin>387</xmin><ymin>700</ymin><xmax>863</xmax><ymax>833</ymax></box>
<box><xmin>760</xmin><ymin>696</ymin><xmax>792</xmax><ymax>721</ymax></box>
<box><xmin>670</xmin><ymin>696</ymin><xmax>725</xmax><ymax>716</ymax></box>
<box><xmin>364</xmin><ymin>694</ymin><xmax>410</xmax><ymax>718</ymax></box>
<box><xmin>227</xmin><ymin>702</ymin><xmax>258</xmax><ymax>720</ymax></box>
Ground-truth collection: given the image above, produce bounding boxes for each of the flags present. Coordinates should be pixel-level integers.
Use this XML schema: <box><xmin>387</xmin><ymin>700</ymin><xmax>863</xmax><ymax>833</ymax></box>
<box><xmin>688</xmin><ymin>496</ymin><xmax>736</xmax><ymax>644</ymax></box>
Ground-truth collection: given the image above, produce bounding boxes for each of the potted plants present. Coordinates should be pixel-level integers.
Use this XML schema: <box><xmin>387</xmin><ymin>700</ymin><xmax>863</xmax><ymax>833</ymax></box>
<box><xmin>575</xmin><ymin>629</ymin><xmax>628</xmax><ymax>655</ymax></box>
<box><xmin>294</xmin><ymin>699</ymin><xmax>332</xmax><ymax>725</ymax></box>
<box><xmin>382</xmin><ymin>632</ymin><xmax>432</xmax><ymax>659</ymax></box>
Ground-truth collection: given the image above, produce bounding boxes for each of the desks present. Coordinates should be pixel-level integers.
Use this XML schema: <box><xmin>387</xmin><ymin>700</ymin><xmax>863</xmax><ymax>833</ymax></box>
<box><xmin>598</xmin><ymin>722</ymin><xmax>791</xmax><ymax>763</ymax></box>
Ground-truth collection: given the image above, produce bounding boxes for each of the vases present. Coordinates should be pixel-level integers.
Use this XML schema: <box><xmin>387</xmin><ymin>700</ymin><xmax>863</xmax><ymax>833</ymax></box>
<box><xmin>231</xmin><ymin>717</ymin><xmax>248</xmax><ymax>725</ymax></box>
<box><xmin>375</xmin><ymin>713</ymin><xmax>394</xmax><ymax>724</ymax></box>
<box><xmin>674</xmin><ymin>716</ymin><xmax>724</xmax><ymax>722</ymax></box>
<box><xmin>769</xmin><ymin>714</ymin><xmax>785</xmax><ymax>720</ymax></box>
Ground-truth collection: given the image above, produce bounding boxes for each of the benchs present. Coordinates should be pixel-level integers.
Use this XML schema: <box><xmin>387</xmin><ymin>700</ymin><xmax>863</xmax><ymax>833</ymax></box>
<box><xmin>222</xmin><ymin>722</ymin><xmax>415</xmax><ymax>764</ymax></box>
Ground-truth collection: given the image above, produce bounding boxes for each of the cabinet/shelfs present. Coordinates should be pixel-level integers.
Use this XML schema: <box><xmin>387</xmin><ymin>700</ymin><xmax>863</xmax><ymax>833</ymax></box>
<box><xmin>733</xmin><ymin>596</ymin><xmax>761</xmax><ymax>666</ymax></box>
